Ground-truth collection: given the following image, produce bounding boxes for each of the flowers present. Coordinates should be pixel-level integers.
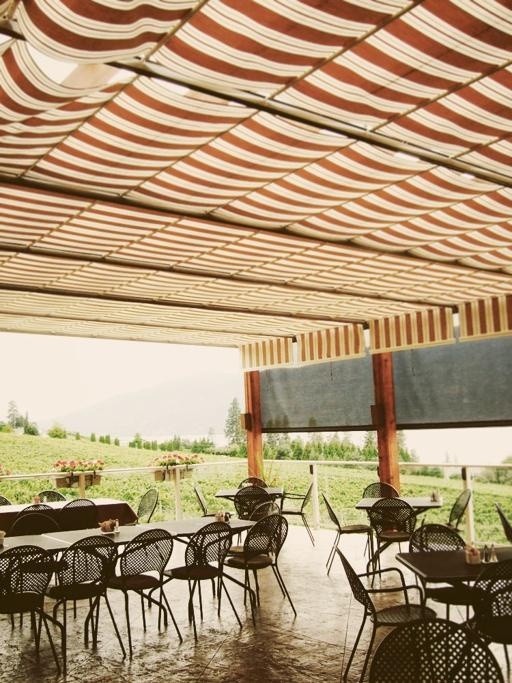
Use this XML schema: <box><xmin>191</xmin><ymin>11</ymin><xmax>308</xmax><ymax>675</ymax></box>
<box><xmin>54</xmin><ymin>459</ymin><xmax>105</xmax><ymax>471</ymax></box>
<box><xmin>154</xmin><ymin>453</ymin><xmax>206</xmax><ymax>466</ymax></box>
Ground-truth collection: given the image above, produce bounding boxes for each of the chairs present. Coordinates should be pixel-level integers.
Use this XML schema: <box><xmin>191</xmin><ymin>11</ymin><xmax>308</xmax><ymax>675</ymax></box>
<box><xmin>0</xmin><ymin>477</ymin><xmax>286</xmax><ymax>551</ymax></box>
<box><xmin>217</xmin><ymin>513</ymin><xmax>297</xmax><ymax>628</ymax></box>
<box><xmin>161</xmin><ymin>521</ymin><xmax>242</xmax><ymax>645</ymax></box>
<box><xmin>0</xmin><ymin>545</ymin><xmax>63</xmax><ymax>672</ymax></box>
<box><xmin>287</xmin><ymin>478</ymin><xmax>512</xmax><ymax>683</ymax></box>
<box><xmin>44</xmin><ymin>536</ymin><xmax>127</xmax><ymax>661</ymax></box>
<box><xmin>100</xmin><ymin>528</ymin><xmax>184</xmax><ymax>658</ymax></box>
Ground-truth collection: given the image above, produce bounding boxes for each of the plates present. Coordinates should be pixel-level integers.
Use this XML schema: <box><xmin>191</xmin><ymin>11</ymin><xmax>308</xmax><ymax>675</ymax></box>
<box><xmin>99</xmin><ymin>527</ymin><xmax>114</xmax><ymax>534</ymax></box>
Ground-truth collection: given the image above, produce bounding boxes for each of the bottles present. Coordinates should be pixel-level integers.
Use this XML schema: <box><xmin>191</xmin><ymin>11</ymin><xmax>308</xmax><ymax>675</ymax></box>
<box><xmin>490</xmin><ymin>543</ymin><xmax>498</xmax><ymax>563</ymax></box>
<box><xmin>214</xmin><ymin>506</ymin><xmax>231</xmax><ymax>524</ymax></box>
<box><xmin>431</xmin><ymin>489</ymin><xmax>439</xmax><ymax>502</ymax></box>
<box><xmin>34</xmin><ymin>494</ymin><xmax>40</xmax><ymax>504</ymax></box>
<box><xmin>43</xmin><ymin>495</ymin><xmax>48</xmax><ymax>505</ymax></box>
<box><xmin>0</xmin><ymin>531</ymin><xmax>6</xmax><ymax>548</ymax></box>
<box><xmin>481</xmin><ymin>544</ymin><xmax>490</xmax><ymax>564</ymax></box>
<box><xmin>115</xmin><ymin>518</ymin><xmax>120</xmax><ymax>533</ymax></box>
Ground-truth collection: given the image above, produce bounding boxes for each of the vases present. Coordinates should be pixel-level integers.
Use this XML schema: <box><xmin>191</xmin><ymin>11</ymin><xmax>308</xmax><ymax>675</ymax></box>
<box><xmin>155</xmin><ymin>468</ymin><xmax>193</xmax><ymax>481</ymax></box>
